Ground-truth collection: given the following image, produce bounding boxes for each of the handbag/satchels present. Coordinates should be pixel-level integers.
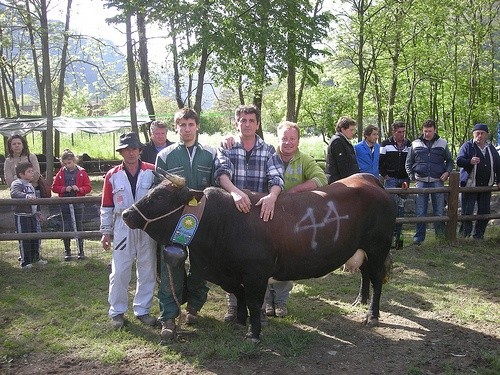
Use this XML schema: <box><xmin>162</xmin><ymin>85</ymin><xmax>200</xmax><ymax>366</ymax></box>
<box><xmin>37</xmin><ymin>175</ymin><xmax>51</xmax><ymax>198</ymax></box>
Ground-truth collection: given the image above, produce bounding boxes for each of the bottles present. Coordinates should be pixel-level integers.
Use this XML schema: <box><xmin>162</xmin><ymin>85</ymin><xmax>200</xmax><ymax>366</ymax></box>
<box><xmin>391</xmin><ymin>232</ymin><xmax>403</xmax><ymax>250</ymax></box>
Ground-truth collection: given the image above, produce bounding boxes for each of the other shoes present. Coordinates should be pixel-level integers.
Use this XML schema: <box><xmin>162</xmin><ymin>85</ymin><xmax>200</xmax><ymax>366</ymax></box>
<box><xmin>474</xmin><ymin>236</ymin><xmax>485</xmax><ymax>244</ymax></box>
<box><xmin>65</xmin><ymin>255</ymin><xmax>70</xmax><ymax>261</ymax></box>
<box><xmin>78</xmin><ymin>255</ymin><xmax>84</xmax><ymax>260</ymax></box>
<box><xmin>390</xmin><ymin>236</ymin><xmax>397</xmax><ymax>248</ymax></box>
<box><xmin>18</xmin><ymin>256</ymin><xmax>22</xmax><ymax>260</ymax></box>
<box><xmin>436</xmin><ymin>234</ymin><xmax>444</xmax><ymax>240</ymax></box>
<box><xmin>458</xmin><ymin>235</ymin><xmax>469</xmax><ymax>243</ymax></box>
<box><xmin>414</xmin><ymin>239</ymin><xmax>423</xmax><ymax>245</ymax></box>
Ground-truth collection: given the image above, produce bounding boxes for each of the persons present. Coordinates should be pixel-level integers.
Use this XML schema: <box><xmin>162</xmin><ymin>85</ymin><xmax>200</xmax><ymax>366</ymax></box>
<box><xmin>140</xmin><ymin>119</ymin><xmax>176</xmax><ymax>289</ymax></box>
<box><xmin>51</xmin><ymin>149</ymin><xmax>92</xmax><ymax>260</ymax></box>
<box><xmin>100</xmin><ymin>133</ymin><xmax>171</xmax><ymax>330</ymax></box>
<box><xmin>9</xmin><ymin>163</ymin><xmax>49</xmax><ymax>271</ymax></box>
<box><xmin>213</xmin><ymin>104</ymin><xmax>285</xmax><ymax>324</ymax></box>
<box><xmin>405</xmin><ymin>119</ymin><xmax>454</xmax><ymax>247</ymax></box>
<box><xmin>325</xmin><ymin>116</ymin><xmax>361</xmax><ymax>185</ymax></box>
<box><xmin>4</xmin><ymin>134</ymin><xmax>41</xmax><ymax>261</ymax></box>
<box><xmin>353</xmin><ymin>124</ymin><xmax>381</xmax><ymax>180</ymax></box>
<box><xmin>379</xmin><ymin>122</ymin><xmax>411</xmax><ymax>250</ymax></box>
<box><xmin>220</xmin><ymin>120</ymin><xmax>328</xmax><ymax>317</ymax></box>
<box><xmin>154</xmin><ymin>108</ymin><xmax>215</xmax><ymax>338</ymax></box>
<box><xmin>457</xmin><ymin>123</ymin><xmax>500</xmax><ymax>241</ymax></box>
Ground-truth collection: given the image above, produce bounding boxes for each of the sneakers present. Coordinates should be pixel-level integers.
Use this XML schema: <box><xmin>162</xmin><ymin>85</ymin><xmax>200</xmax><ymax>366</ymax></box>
<box><xmin>275</xmin><ymin>303</ymin><xmax>287</xmax><ymax>317</ymax></box>
<box><xmin>266</xmin><ymin>303</ymin><xmax>274</xmax><ymax>316</ymax></box>
<box><xmin>186</xmin><ymin>307</ymin><xmax>199</xmax><ymax>323</ymax></box>
<box><xmin>21</xmin><ymin>263</ymin><xmax>33</xmax><ymax>270</ymax></box>
<box><xmin>112</xmin><ymin>314</ymin><xmax>125</xmax><ymax>328</ymax></box>
<box><xmin>137</xmin><ymin>315</ymin><xmax>156</xmax><ymax>326</ymax></box>
<box><xmin>34</xmin><ymin>259</ymin><xmax>48</xmax><ymax>265</ymax></box>
<box><xmin>227</xmin><ymin>305</ymin><xmax>238</xmax><ymax>322</ymax></box>
<box><xmin>260</xmin><ymin>311</ymin><xmax>267</xmax><ymax>323</ymax></box>
<box><xmin>161</xmin><ymin>318</ymin><xmax>176</xmax><ymax>339</ymax></box>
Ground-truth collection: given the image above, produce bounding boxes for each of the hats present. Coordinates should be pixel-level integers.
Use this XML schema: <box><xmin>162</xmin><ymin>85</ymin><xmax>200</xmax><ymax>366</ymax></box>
<box><xmin>472</xmin><ymin>124</ymin><xmax>488</xmax><ymax>133</ymax></box>
<box><xmin>116</xmin><ymin>132</ymin><xmax>145</xmax><ymax>151</ymax></box>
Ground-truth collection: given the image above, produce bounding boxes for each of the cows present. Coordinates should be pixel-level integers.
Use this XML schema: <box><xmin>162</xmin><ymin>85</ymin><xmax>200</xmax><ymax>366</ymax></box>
<box><xmin>122</xmin><ymin>167</ymin><xmax>396</xmax><ymax>342</ymax></box>
